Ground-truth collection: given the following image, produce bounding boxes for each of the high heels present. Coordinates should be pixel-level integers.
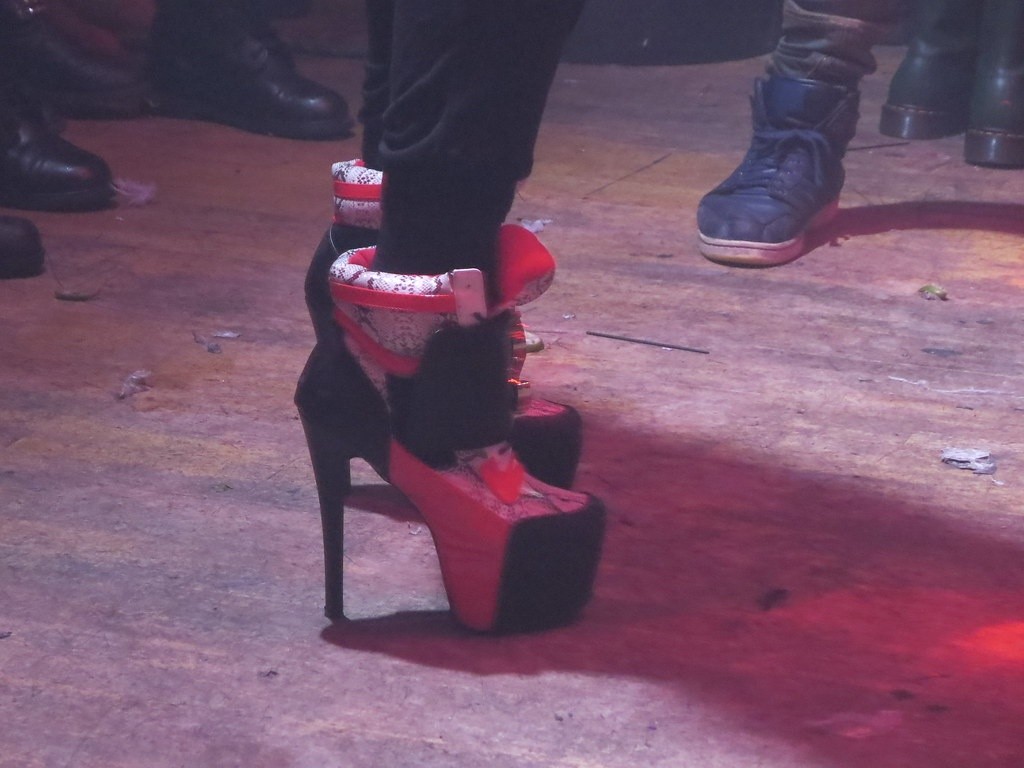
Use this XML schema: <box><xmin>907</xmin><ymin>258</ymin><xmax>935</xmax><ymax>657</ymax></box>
<box><xmin>293</xmin><ymin>223</ymin><xmax>607</xmax><ymax>633</ymax></box>
<box><xmin>304</xmin><ymin>156</ymin><xmax>582</xmax><ymax>495</ymax></box>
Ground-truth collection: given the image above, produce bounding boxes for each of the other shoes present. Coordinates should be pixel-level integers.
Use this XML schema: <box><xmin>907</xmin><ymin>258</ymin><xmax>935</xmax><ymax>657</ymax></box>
<box><xmin>964</xmin><ymin>57</ymin><xmax>1024</xmax><ymax>166</ymax></box>
<box><xmin>879</xmin><ymin>35</ymin><xmax>975</xmax><ymax>140</ymax></box>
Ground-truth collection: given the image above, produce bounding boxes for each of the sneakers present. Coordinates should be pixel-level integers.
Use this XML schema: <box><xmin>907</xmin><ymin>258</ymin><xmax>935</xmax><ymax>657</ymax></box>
<box><xmin>697</xmin><ymin>73</ymin><xmax>860</xmax><ymax>265</ymax></box>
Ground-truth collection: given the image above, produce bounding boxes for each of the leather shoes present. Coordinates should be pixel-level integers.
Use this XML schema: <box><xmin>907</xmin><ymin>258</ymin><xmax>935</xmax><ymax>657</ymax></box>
<box><xmin>0</xmin><ymin>13</ymin><xmax>146</xmax><ymax>119</ymax></box>
<box><xmin>0</xmin><ymin>112</ymin><xmax>118</xmax><ymax>210</ymax></box>
<box><xmin>139</xmin><ymin>22</ymin><xmax>356</xmax><ymax>138</ymax></box>
<box><xmin>0</xmin><ymin>216</ymin><xmax>46</xmax><ymax>279</ymax></box>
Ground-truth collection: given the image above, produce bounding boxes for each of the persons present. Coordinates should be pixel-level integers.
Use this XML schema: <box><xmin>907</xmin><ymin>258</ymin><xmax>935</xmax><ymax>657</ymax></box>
<box><xmin>293</xmin><ymin>0</ymin><xmax>604</xmax><ymax>635</ymax></box>
<box><xmin>0</xmin><ymin>0</ymin><xmax>354</xmax><ymax>281</ymax></box>
<box><xmin>695</xmin><ymin>0</ymin><xmax>1024</xmax><ymax>268</ymax></box>
